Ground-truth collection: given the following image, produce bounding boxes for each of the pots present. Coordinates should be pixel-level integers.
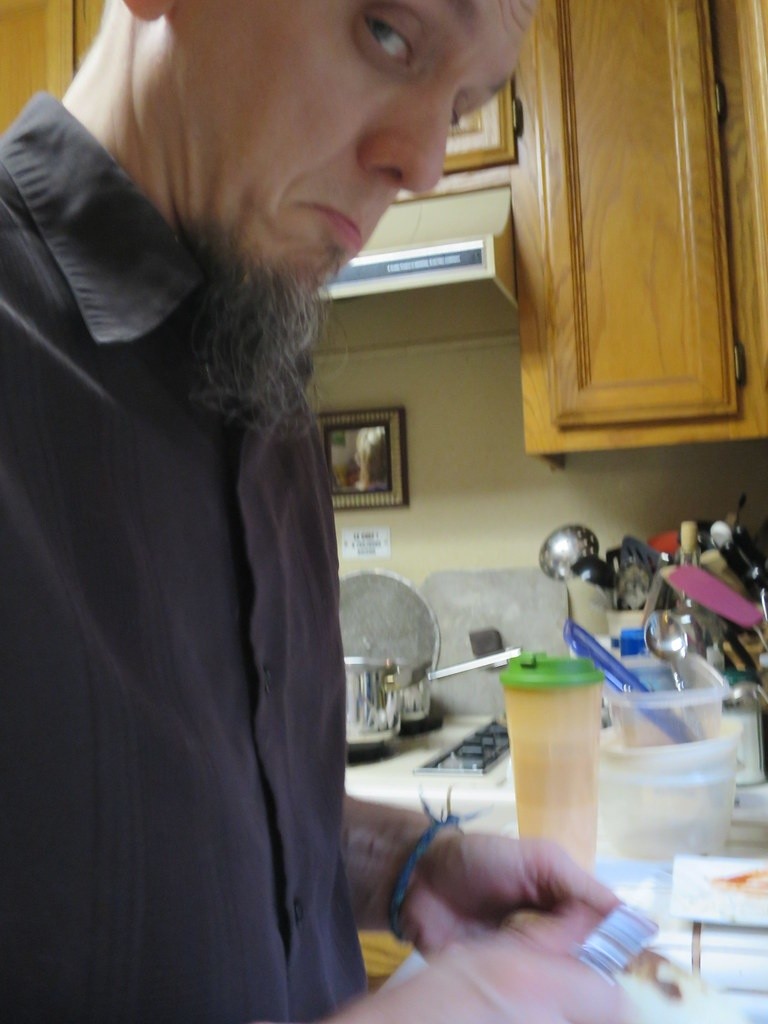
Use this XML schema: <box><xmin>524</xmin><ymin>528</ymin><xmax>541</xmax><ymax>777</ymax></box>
<box><xmin>345</xmin><ymin>656</ymin><xmax>433</xmax><ymax>744</ymax></box>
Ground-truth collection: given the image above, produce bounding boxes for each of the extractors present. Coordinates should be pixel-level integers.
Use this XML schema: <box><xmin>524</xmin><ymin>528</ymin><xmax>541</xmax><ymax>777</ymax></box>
<box><xmin>311</xmin><ymin>185</ymin><xmax>518</xmax><ymax>368</ymax></box>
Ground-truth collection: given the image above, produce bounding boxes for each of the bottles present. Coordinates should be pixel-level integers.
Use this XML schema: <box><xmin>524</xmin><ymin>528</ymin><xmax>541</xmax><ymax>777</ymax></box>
<box><xmin>673</xmin><ymin>519</ymin><xmax>724</xmax><ymax>678</ymax></box>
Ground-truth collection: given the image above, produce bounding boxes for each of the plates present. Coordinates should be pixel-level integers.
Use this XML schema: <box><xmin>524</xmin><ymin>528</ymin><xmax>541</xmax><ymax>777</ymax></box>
<box><xmin>669</xmin><ymin>851</ymin><xmax>768</xmax><ymax>926</ymax></box>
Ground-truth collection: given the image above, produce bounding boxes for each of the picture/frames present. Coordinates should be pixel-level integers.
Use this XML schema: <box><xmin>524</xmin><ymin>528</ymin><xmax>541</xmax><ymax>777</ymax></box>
<box><xmin>319</xmin><ymin>406</ymin><xmax>411</xmax><ymax>511</ymax></box>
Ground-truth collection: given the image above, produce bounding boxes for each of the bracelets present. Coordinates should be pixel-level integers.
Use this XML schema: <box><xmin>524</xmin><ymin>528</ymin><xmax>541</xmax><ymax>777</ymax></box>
<box><xmin>387</xmin><ymin>822</ymin><xmax>462</xmax><ymax>938</ymax></box>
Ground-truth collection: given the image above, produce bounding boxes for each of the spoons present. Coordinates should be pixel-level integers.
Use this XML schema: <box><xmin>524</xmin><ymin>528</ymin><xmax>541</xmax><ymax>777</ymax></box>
<box><xmin>644</xmin><ymin>609</ymin><xmax>704</xmax><ymax>739</ymax></box>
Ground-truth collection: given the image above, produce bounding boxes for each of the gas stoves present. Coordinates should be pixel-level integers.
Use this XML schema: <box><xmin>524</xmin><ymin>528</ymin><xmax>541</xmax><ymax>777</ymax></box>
<box><xmin>346</xmin><ymin>710</ymin><xmax>511</xmax><ymax>778</ymax></box>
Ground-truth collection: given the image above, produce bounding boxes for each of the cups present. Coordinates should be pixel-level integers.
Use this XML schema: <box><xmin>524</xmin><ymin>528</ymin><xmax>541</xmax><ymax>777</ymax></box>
<box><xmin>500</xmin><ymin>652</ymin><xmax>607</xmax><ymax>869</ymax></box>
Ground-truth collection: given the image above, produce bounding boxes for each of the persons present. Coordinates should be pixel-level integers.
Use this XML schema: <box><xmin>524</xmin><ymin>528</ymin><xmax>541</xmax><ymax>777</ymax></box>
<box><xmin>0</xmin><ymin>1</ymin><xmax>686</xmax><ymax>1022</ymax></box>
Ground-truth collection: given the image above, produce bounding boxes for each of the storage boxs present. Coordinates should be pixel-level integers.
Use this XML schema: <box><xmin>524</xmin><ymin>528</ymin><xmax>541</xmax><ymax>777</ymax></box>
<box><xmin>600</xmin><ymin>648</ymin><xmax>731</xmax><ymax>745</ymax></box>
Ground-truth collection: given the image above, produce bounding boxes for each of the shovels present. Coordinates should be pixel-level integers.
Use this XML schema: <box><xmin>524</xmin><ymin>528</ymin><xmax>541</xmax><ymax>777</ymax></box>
<box><xmin>669</xmin><ymin>565</ymin><xmax>768</xmax><ymax>653</ymax></box>
<box><xmin>606</xmin><ymin>547</ymin><xmax>621</xmax><ymax>586</ymax></box>
<box><xmin>619</xmin><ymin>536</ymin><xmax>678</xmax><ymax>601</ymax></box>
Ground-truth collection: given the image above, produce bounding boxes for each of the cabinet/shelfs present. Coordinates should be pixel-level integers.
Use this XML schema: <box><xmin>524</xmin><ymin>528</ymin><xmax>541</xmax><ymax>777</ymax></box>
<box><xmin>0</xmin><ymin>0</ymin><xmax>768</xmax><ymax>471</ymax></box>
